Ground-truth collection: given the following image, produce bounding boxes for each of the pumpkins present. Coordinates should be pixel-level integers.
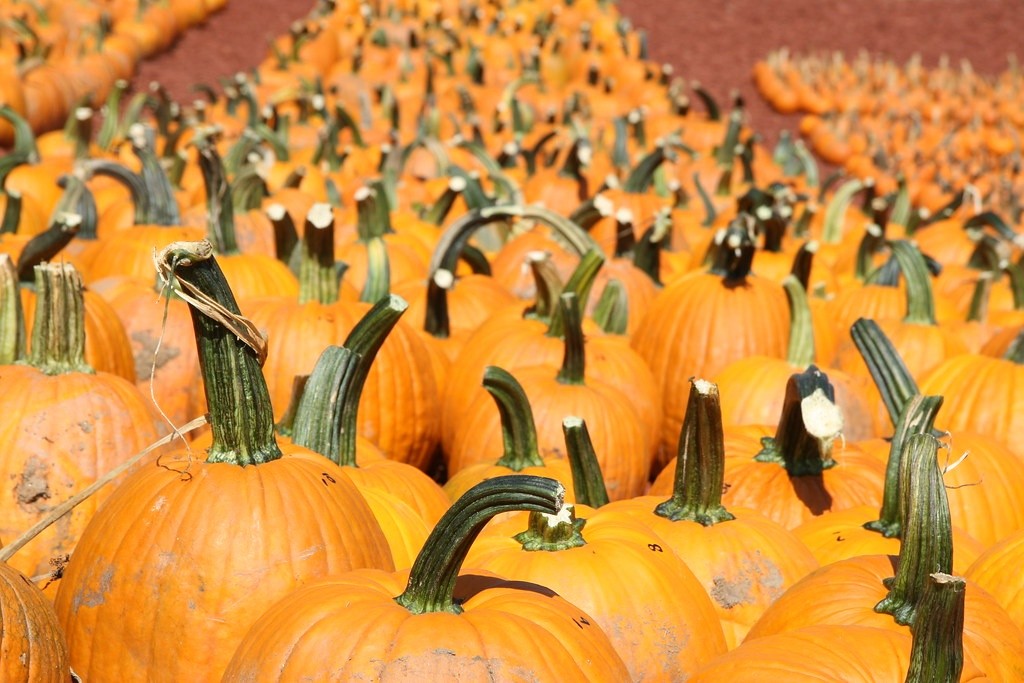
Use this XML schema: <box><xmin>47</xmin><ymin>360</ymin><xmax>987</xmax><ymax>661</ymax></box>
<box><xmin>0</xmin><ymin>0</ymin><xmax>1024</xmax><ymax>683</ymax></box>
